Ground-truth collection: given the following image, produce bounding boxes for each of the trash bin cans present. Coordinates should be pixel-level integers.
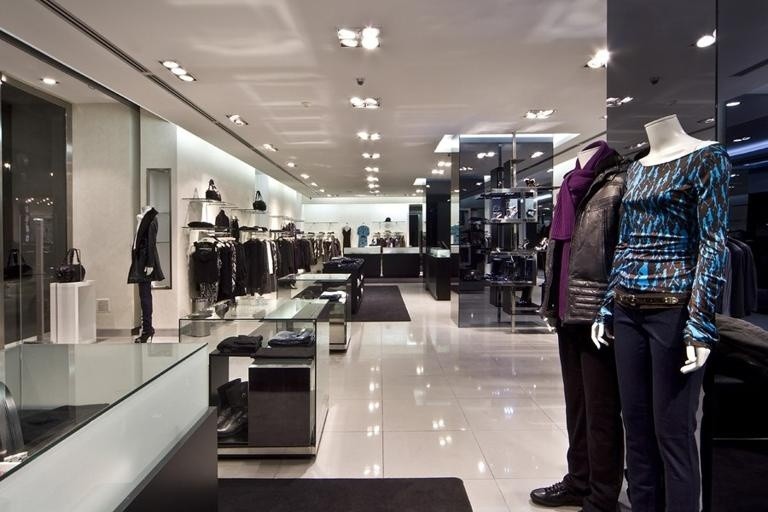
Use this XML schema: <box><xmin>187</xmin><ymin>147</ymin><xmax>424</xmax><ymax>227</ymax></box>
<box><xmin>189</xmin><ymin>297</ymin><xmax>209</xmax><ymax>337</ymax></box>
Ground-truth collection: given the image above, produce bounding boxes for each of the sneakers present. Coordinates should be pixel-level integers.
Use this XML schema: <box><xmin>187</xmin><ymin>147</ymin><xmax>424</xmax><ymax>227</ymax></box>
<box><xmin>531</xmin><ymin>480</ymin><xmax>583</xmax><ymax>507</ymax></box>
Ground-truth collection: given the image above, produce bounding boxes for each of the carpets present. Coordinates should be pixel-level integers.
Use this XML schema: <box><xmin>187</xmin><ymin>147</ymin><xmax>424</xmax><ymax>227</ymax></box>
<box><xmin>258</xmin><ymin>285</ymin><xmax>411</xmax><ymax>321</ymax></box>
<box><xmin>218</xmin><ymin>479</ymin><xmax>473</xmax><ymax>512</ymax></box>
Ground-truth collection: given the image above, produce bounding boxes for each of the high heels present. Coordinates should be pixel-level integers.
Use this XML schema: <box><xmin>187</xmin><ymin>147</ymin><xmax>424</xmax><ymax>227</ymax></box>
<box><xmin>134</xmin><ymin>327</ymin><xmax>155</xmax><ymax>343</ymax></box>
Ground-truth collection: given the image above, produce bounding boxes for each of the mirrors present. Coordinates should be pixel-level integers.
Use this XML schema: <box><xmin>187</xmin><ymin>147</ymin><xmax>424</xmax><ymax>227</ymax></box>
<box><xmin>450</xmin><ymin>133</ymin><xmax>553</xmax><ymax>328</ymax></box>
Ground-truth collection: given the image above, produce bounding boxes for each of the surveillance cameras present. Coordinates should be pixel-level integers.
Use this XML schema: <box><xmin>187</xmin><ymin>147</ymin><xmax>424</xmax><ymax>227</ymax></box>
<box><xmin>356</xmin><ymin>77</ymin><xmax>365</xmax><ymax>86</ymax></box>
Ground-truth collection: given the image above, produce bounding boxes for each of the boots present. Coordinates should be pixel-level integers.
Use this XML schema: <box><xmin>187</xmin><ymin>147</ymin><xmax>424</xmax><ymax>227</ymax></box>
<box><xmin>216</xmin><ymin>378</ymin><xmax>248</xmax><ymax>437</ymax></box>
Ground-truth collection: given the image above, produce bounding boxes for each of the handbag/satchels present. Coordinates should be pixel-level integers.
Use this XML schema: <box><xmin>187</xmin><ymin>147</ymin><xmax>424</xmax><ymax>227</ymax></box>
<box><xmin>53</xmin><ymin>248</ymin><xmax>85</xmax><ymax>282</ymax></box>
<box><xmin>4</xmin><ymin>249</ymin><xmax>34</xmax><ymax>281</ymax></box>
<box><xmin>215</xmin><ymin>210</ymin><xmax>230</xmax><ymax>227</ymax></box>
<box><xmin>253</xmin><ymin>191</ymin><xmax>266</xmax><ymax>210</ymax></box>
<box><xmin>207</xmin><ymin>180</ymin><xmax>221</xmax><ymax>201</ymax></box>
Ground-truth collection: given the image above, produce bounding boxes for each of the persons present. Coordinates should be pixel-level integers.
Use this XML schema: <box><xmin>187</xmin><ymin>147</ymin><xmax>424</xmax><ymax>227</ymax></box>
<box><xmin>589</xmin><ymin>112</ymin><xmax>732</xmax><ymax>512</ymax></box>
<box><xmin>528</xmin><ymin>139</ymin><xmax>632</xmax><ymax>512</ymax></box>
<box><xmin>124</xmin><ymin>204</ymin><xmax>164</xmax><ymax>344</ymax></box>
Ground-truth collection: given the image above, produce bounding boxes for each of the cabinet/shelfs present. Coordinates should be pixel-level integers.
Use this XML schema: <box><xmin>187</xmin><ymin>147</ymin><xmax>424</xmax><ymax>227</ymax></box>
<box><xmin>181</xmin><ymin>197</ymin><xmax>338</xmax><ymax>236</ymax></box>
<box><xmin>371</xmin><ymin>221</ymin><xmax>406</xmax><ymax>236</ymax></box>
<box><xmin>177</xmin><ymin>296</ymin><xmax>331</xmax><ymax>460</ymax></box>
<box><xmin>474</xmin><ymin>166</ymin><xmax>505</xmax><ymax>323</ymax></box>
<box><xmin>485</xmin><ymin>159</ymin><xmax>561</xmax><ymax>334</ymax></box>
<box><xmin>49</xmin><ymin>280</ymin><xmax>96</xmax><ymax>345</ymax></box>
<box><xmin>322</xmin><ymin>259</ymin><xmax>365</xmax><ymax>314</ymax></box>
<box><xmin>0</xmin><ymin>338</ymin><xmax>219</xmax><ymax>512</ymax></box>
<box><xmin>276</xmin><ymin>274</ymin><xmax>353</xmax><ymax>353</ymax></box>
